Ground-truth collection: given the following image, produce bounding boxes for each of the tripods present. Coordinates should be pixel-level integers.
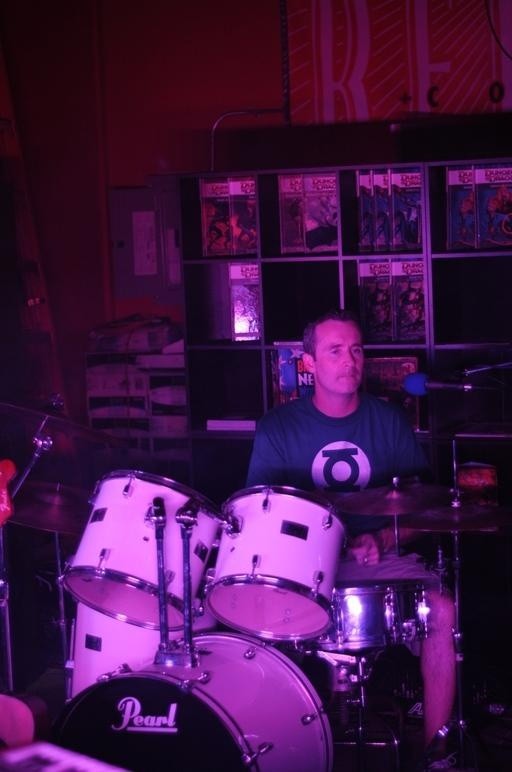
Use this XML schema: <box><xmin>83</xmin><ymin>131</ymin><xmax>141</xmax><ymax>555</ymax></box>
<box><xmin>331</xmin><ymin>655</ymin><xmax>402</xmax><ymax>772</ymax></box>
<box><xmin>413</xmin><ymin>442</ymin><xmax>510</xmax><ymax>772</ymax></box>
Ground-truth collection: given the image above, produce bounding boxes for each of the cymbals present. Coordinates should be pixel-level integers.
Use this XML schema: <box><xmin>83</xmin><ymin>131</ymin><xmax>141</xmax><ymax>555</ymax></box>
<box><xmin>5</xmin><ymin>479</ymin><xmax>93</xmax><ymax>536</ymax></box>
<box><xmin>389</xmin><ymin>502</ymin><xmax>511</xmax><ymax>533</ymax></box>
<box><xmin>317</xmin><ymin>485</ymin><xmax>452</xmax><ymax>515</ymax></box>
<box><xmin>0</xmin><ymin>404</ymin><xmax>144</xmax><ymax>470</ymax></box>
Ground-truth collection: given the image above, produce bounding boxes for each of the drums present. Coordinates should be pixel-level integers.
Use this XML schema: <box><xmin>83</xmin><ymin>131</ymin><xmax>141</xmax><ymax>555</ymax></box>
<box><xmin>207</xmin><ymin>485</ymin><xmax>347</xmax><ymax>640</ymax></box>
<box><xmin>54</xmin><ymin>632</ymin><xmax>333</xmax><ymax>771</ymax></box>
<box><xmin>69</xmin><ymin>601</ymin><xmax>214</xmax><ymax>702</ymax></box>
<box><xmin>314</xmin><ymin>646</ymin><xmax>414</xmax><ymax>718</ymax></box>
<box><xmin>382</xmin><ymin>580</ymin><xmax>429</xmax><ymax>645</ymax></box>
<box><xmin>62</xmin><ymin>469</ymin><xmax>224</xmax><ymax>631</ymax></box>
<box><xmin>308</xmin><ymin>585</ymin><xmax>385</xmax><ymax>650</ymax></box>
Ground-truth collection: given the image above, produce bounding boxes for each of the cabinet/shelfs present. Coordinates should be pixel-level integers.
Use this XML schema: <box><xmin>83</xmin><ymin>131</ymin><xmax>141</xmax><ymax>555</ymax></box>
<box><xmin>176</xmin><ymin>160</ymin><xmax>511</xmax><ymax>480</ymax></box>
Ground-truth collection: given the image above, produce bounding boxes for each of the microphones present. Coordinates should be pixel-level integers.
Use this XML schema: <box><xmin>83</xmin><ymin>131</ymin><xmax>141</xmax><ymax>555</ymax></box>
<box><xmin>404</xmin><ymin>374</ymin><xmax>472</xmax><ymax>398</ymax></box>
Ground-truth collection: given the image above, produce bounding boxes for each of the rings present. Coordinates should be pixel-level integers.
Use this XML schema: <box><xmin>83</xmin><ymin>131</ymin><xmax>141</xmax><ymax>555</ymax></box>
<box><xmin>364</xmin><ymin>558</ymin><xmax>368</xmax><ymax>563</ymax></box>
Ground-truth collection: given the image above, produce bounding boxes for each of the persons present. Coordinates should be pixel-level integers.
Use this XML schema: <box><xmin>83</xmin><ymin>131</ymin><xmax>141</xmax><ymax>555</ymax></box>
<box><xmin>245</xmin><ymin>310</ymin><xmax>461</xmax><ymax>772</ymax></box>
